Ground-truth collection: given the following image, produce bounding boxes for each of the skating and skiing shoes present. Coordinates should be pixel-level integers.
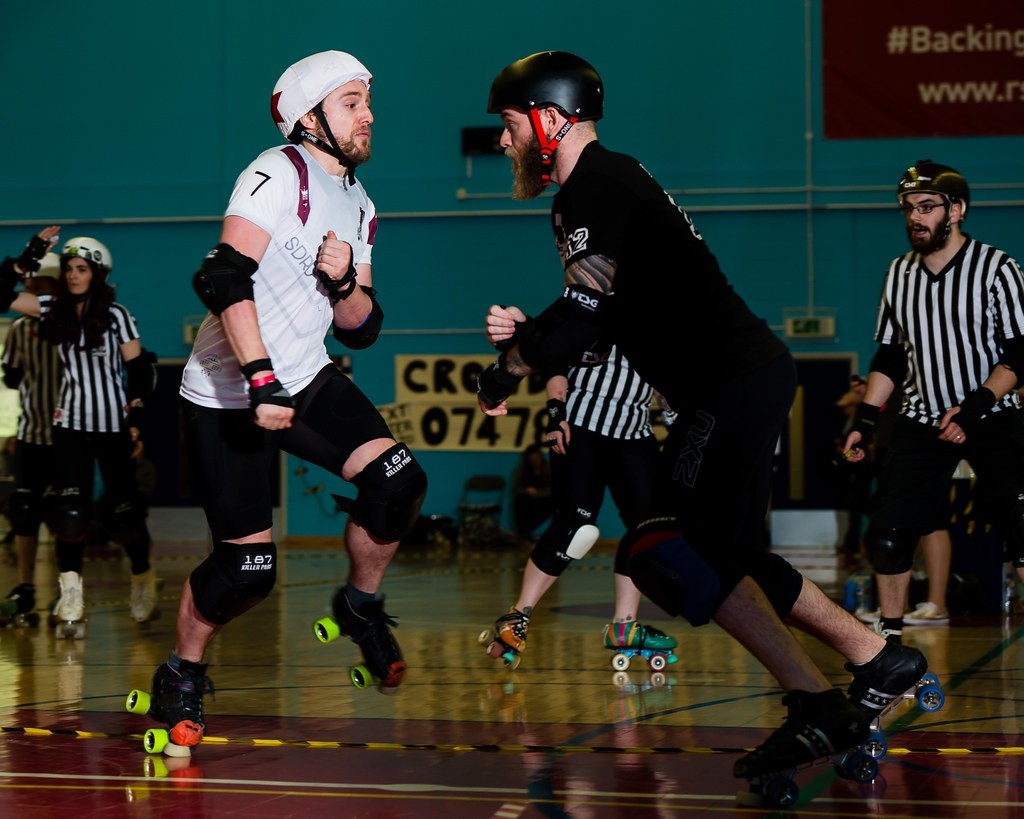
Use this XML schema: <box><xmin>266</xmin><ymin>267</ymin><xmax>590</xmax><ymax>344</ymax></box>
<box><xmin>602</xmin><ymin>618</ymin><xmax>678</xmax><ymax>672</ymax></box>
<box><xmin>732</xmin><ymin>690</ymin><xmax>878</xmax><ymax>807</ymax></box>
<box><xmin>478</xmin><ymin>604</ymin><xmax>530</xmax><ymax>671</ymax></box>
<box><xmin>128</xmin><ymin>565</ymin><xmax>164</xmax><ymax>629</ymax></box>
<box><xmin>847</xmin><ymin>640</ymin><xmax>945</xmax><ymax>760</ymax></box>
<box><xmin>125</xmin><ymin>649</ymin><xmax>215</xmax><ymax>758</ymax></box>
<box><xmin>0</xmin><ymin>586</ymin><xmax>40</xmax><ymax>629</ymax></box>
<box><xmin>49</xmin><ymin>570</ymin><xmax>86</xmax><ymax>641</ymax></box>
<box><xmin>125</xmin><ymin>755</ymin><xmax>204</xmax><ymax>818</ymax></box>
<box><xmin>313</xmin><ymin>584</ymin><xmax>406</xmax><ymax>694</ymax></box>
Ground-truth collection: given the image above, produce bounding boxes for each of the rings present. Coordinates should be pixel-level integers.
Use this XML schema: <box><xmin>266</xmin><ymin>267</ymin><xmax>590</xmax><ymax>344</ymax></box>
<box><xmin>956</xmin><ymin>435</ymin><xmax>960</xmax><ymax>439</ymax></box>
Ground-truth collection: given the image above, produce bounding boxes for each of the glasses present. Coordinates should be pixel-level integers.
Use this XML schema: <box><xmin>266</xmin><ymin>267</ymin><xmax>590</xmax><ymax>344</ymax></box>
<box><xmin>903</xmin><ymin>201</ymin><xmax>944</xmax><ymax>214</ymax></box>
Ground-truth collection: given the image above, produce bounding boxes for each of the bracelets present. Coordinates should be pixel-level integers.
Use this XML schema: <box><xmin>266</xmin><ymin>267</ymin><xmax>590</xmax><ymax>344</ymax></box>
<box><xmin>251</xmin><ymin>373</ymin><xmax>274</xmax><ymax>387</ymax></box>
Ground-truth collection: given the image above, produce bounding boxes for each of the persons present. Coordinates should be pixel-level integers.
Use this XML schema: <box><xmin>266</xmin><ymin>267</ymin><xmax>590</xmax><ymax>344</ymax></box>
<box><xmin>835</xmin><ymin>163</ymin><xmax>1024</xmax><ymax>672</ymax></box>
<box><xmin>1</xmin><ymin>224</ymin><xmax>162</xmax><ymax>623</ymax></box>
<box><xmin>477</xmin><ymin>48</ymin><xmax>927</xmax><ymax>782</ymax></box>
<box><xmin>150</xmin><ymin>47</ymin><xmax>430</xmax><ymax>744</ymax></box>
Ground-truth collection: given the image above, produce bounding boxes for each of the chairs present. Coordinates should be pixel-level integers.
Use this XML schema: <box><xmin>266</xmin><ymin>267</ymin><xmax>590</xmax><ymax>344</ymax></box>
<box><xmin>457</xmin><ymin>471</ymin><xmax>507</xmax><ymax>552</ymax></box>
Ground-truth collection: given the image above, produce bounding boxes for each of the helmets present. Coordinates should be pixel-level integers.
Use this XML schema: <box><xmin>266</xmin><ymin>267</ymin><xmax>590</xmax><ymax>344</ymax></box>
<box><xmin>896</xmin><ymin>161</ymin><xmax>970</xmax><ymax>223</ymax></box>
<box><xmin>62</xmin><ymin>236</ymin><xmax>112</xmax><ymax>270</ymax></box>
<box><xmin>271</xmin><ymin>50</ymin><xmax>372</xmax><ymax>142</ymax></box>
<box><xmin>25</xmin><ymin>252</ymin><xmax>61</xmax><ymax>279</ymax></box>
<box><xmin>487</xmin><ymin>50</ymin><xmax>604</xmax><ymax>122</ymax></box>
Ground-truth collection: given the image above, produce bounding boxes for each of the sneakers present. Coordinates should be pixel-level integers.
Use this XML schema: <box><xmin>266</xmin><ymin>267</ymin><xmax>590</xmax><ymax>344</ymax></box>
<box><xmin>901</xmin><ymin>602</ymin><xmax>950</xmax><ymax>625</ymax></box>
<box><xmin>857</xmin><ymin>607</ymin><xmax>883</xmax><ymax>624</ymax></box>
